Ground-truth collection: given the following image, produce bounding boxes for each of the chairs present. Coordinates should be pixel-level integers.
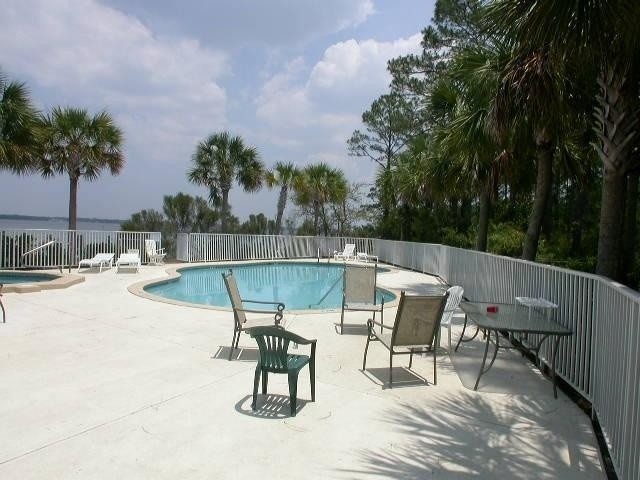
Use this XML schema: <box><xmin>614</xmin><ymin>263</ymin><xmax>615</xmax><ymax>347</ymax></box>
<box><xmin>421</xmin><ymin>285</ymin><xmax>465</xmax><ymax>359</ymax></box>
<box><xmin>115</xmin><ymin>249</ymin><xmax>141</xmax><ymax>274</ymax></box>
<box><xmin>145</xmin><ymin>239</ymin><xmax>167</xmax><ymax>267</ymax></box>
<box><xmin>357</xmin><ymin>252</ymin><xmax>379</xmax><ymax>264</ymax></box>
<box><xmin>333</xmin><ymin>261</ymin><xmax>385</xmax><ymax>336</ymax></box>
<box><xmin>334</xmin><ymin>244</ymin><xmax>356</xmax><ymax>261</ymax></box>
<box><xmin>220</xmin><ymin>268</ymin><xmax>286</xmax><ymax>362</ymax></box>
<box><xmin>362</xmin><ymin>289</ymin><xmax>451</xmax><ymax>389</ymax></box>
<box><xmin>77</xmin><ymin>252</ymin><xmax>116</xmax><ymax>273</ymax></box>
<box><xmin>244</xmin><ymin>325</ymin><xmax>318</xmax><ymax>418</ymax></box>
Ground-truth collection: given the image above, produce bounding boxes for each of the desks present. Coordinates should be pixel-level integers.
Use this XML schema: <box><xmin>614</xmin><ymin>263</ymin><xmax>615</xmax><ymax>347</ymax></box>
<box><xmin>453</xmin><ymin>296</ymin><xmax>574</xmax><ymax>400</ymax></box>
